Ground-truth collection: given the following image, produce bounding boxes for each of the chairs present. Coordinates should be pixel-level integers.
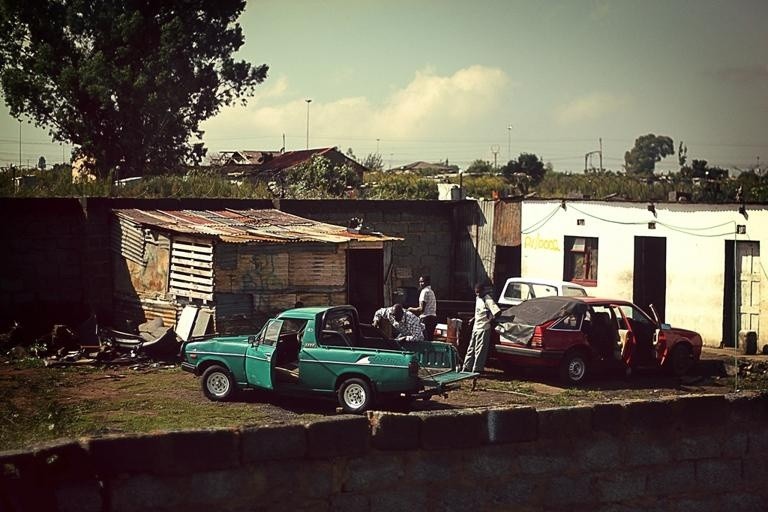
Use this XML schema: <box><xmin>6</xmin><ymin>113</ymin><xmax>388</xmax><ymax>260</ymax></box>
<box><xmin>590</xmin><ymin>312</ymin><xmax>610</xmax><ymax>328</ymax></box>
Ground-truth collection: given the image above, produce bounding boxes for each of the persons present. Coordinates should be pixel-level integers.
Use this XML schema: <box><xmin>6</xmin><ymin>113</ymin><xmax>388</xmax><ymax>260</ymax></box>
<box><xmin>407</xmin><ymin>276</ymin><xmax>438</xmax><ymax>342</ymax></box>
<box><xmin>371</xmin><ymin>302</ymin><xmax>425</xmax><ymax>341</ymax></box>
<box><xmin>287</xmin><ymin>301</ymin><xmax>304</xmax><ymax>333</ymax></box>
<box><xmin>460</xmin><ymin>283</ymin><xmax>502</xmax><ymax>374</ymax></box>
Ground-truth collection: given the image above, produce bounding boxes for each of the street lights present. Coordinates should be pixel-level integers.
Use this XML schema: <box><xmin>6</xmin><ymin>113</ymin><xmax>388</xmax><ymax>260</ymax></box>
<box><xmin>505</xmin><ymin>123</ymin><xmax>510</xmax><ymax>159</ymax></box>
<box><xmin>17</xmin><ymin>116</ymin><xmax>24</xmax><ymax>171</ymax></box>
<box><xmin>304</xmin><ymin>95</ymin><xmax>311</xmax><ymax>150</ymax></box>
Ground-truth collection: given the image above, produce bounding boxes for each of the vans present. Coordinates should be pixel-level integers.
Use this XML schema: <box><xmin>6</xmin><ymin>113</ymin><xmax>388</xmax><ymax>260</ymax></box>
<box><xmin>497</xmin><ymin>278</ymin><xmax>589</xmax><ymax>306</ymax></box>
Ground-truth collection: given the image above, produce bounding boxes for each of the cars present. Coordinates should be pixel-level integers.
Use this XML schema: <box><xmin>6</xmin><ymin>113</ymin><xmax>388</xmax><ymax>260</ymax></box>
<box><xmin>181</xmin><ymin>305</ymin><xmax>479</xmax><ymax>414</ymax></box>
<box><xmin>488</xmin><ymin>295</ymin><xmax>703</xmax><ymax>384</ymax></box>
